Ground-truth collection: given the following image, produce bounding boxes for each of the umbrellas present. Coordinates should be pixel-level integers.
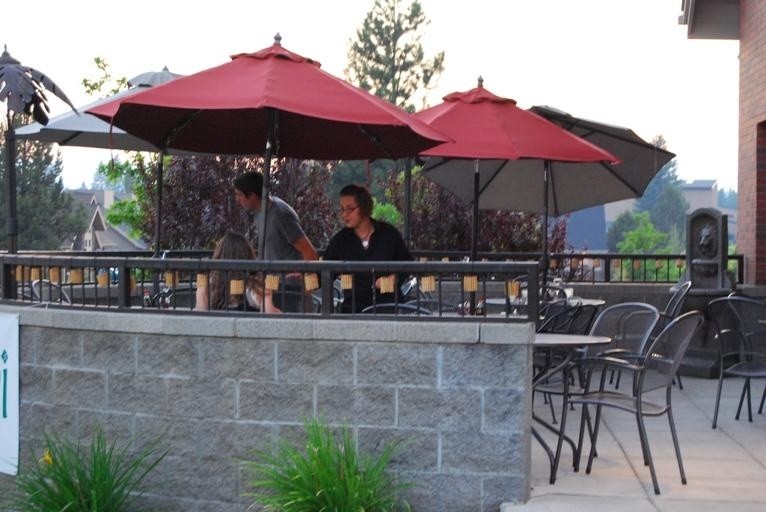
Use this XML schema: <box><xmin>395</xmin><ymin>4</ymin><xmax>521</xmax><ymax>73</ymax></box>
<box><xmin>83</xmin><ymin>32</ymin><xmax>457</xmax><ymax>258</ymax></box>
<box><xmin>12</xmin><ymin>65</ymin><xmax>213</xmax><ymax>294</ymax></box>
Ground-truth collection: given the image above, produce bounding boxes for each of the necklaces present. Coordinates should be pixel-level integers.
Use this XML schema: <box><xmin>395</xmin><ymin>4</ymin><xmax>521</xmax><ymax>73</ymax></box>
<box><xmin>357</xmin><ymin>221</ymin><xmax>372</xmax><ymax>248</ymax></box>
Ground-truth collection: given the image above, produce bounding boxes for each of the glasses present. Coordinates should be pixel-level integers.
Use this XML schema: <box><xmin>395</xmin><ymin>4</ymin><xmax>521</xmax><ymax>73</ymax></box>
<box><xmin>339</xmin><ymin>207</ymin><xmax>358</xmax><ymax>213</ymax></box>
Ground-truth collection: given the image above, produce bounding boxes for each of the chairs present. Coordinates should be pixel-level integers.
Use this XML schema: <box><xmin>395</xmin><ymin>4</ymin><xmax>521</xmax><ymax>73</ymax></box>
<box><xmin>31</xmin><ymin>279</ymin><xmax>72</xmax><ymax>304</ymax></box>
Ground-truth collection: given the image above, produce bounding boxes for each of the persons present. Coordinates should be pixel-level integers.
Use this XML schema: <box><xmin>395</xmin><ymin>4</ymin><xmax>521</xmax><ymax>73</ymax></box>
<box><xmin>233</xmin><ymin>170</ymin><xmax>319</xmax><ymax>283</ymax></box>
<box><xmin>196</xmin><ymin>229</ymin><xmax>282</xmax><ymax>314</ymax></box>
<box><xmin>284</xmin><ymin>183</ymin><xmax>411</xmax><ymax>312</ymax></box>
